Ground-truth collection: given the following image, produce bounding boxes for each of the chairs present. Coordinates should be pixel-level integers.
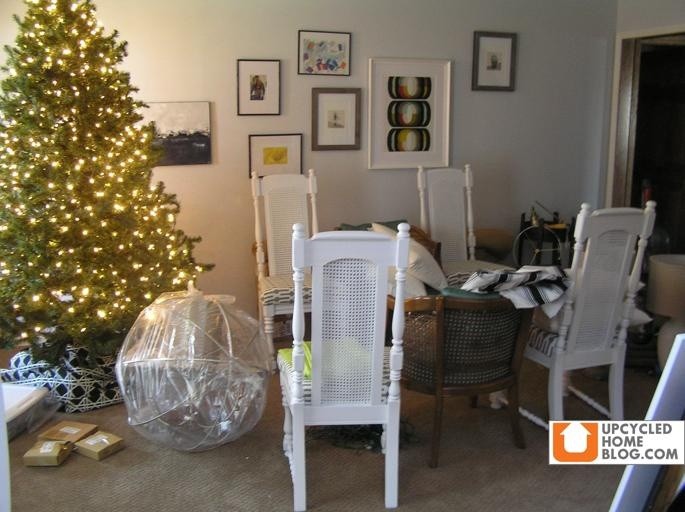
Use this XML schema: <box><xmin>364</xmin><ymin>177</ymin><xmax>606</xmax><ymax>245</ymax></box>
<box><xmin>248</xmin><ymin>166</ymin><xmax>659</xmax><ymax>510</ymax></box>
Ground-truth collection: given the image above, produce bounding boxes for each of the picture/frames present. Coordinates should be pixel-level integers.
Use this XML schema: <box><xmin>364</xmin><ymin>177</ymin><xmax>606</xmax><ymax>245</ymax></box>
<box><xmin>233</xmin><ymin>29</ymin><xmax>518</xmax><ymax>179</ymax></box>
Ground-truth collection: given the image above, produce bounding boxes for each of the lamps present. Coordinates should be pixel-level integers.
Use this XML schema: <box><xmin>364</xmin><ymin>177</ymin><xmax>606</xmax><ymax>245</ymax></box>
<box><xmin>650</xmin><ymin>251</ymin><xmax>684</xmax><ymax>371</ymax></box>
